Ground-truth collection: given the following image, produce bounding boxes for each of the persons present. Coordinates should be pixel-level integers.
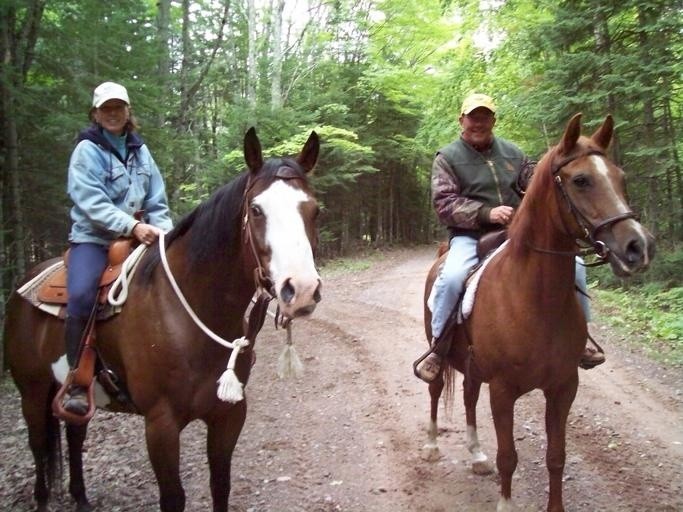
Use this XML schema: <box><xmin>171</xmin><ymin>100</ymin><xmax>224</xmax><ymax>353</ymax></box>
<box><xmin>57</xmin><ymin>82</ymin><xmax>176</xmax><ymax>416</ymax></box>
<box><xmin>418</xmin><ymin>92</ymin><xmax>605</xmax><ymax>383</ymax></box>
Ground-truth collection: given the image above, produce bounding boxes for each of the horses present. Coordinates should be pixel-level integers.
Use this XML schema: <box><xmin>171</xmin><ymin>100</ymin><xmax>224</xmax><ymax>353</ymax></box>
<box><xmin>419</xmin><ymin>109</ymin><xmax>658</xmax><ymax>512</ymax></box>
<box><xmin>0</xmin><ymin>126</ymin><xmax>324</xmax><ymax>512</ymax></box>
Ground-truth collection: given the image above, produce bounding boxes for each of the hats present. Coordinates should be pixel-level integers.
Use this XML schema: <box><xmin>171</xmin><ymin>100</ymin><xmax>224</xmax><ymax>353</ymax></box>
<box><xmin>92</xmin><ymin>82</ymin><xmax>130</xmax><ymax>109</ymax></box>
<box><xmin>461</xmin><ymin>94</ymin><xmax>496</xmax><ymax>115</ymax></box>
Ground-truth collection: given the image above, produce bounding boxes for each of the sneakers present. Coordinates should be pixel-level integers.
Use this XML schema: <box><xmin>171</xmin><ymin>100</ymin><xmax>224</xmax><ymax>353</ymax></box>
<box><xmin>63</xmin><ymin>390</ymin><xmax>89</xmax><ymax>414</ymax></box>
<box><xmin>584</xmin><ymin>343</ymin><xmax>605</xmax><ymax>365</ymax></box>
<box><xmin>418</xmin><ymin>352</ymin><xmax>441</xmax><ymax>382</ymax></box>
